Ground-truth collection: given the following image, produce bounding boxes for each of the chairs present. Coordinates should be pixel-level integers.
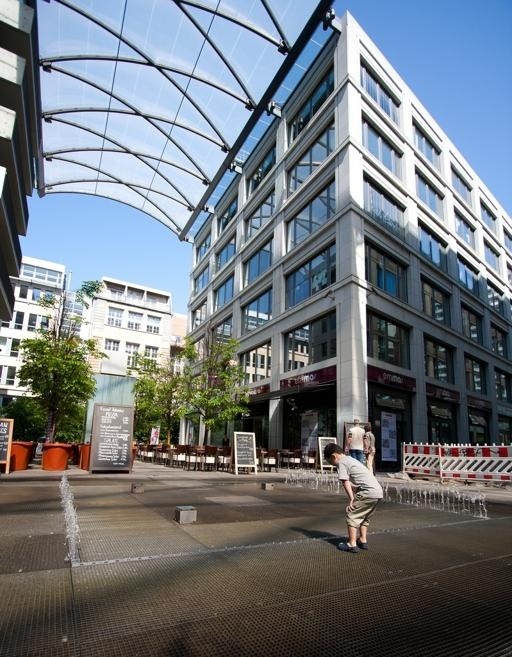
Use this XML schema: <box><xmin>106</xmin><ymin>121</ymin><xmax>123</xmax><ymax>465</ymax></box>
<box><xmin>138</xmin><ymin>442</ymin><xmax>317</xmax><ymax>474</ymax></box>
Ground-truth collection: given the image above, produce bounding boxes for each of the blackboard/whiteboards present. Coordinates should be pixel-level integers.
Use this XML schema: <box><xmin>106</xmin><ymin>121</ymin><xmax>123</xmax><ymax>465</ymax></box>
<box><xmin>234</xmin><ymin>432</ymin><xmax>257</xmax><ymax>467</ymax></box>
<box><xmin>318</xmin><ymin>437</ymin><xmax>336</xmax><ymax>467</ymax></box>
<box><xmin>0</xmin><ymin>418</ymin><xmax>14</xmax><ymax>464</ymax></box>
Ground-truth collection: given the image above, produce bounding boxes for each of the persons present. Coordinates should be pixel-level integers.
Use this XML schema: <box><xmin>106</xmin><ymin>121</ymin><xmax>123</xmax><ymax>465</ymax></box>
<box><xmin>364</xmin><ymin>423</ymin><xmax>378</xmax><ymax>475</ymax></box>
<box><xmin>347</xmin><ymin>418</ymin><xmax>366</xmax><ymax>462</ymax></box>
<box><xmin>325</xmin><ymin>443</ymin><xmax>383</xmax><ymax>552</ymax></box>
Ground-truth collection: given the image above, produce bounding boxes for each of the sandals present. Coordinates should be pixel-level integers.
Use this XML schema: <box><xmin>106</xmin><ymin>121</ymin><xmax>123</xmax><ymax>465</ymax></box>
<box><xmin>356</xmin><ymin>538</ymin><xmax>369</xmax><ymax>549</ymax></box>
<box><xmin>338</xmin><ymin>541</ymin><xmax>359</xmax><ymax>553</ymax></box>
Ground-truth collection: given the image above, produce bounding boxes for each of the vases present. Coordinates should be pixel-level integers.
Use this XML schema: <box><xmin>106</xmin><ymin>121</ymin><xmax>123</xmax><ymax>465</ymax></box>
<box><xmin>10</xmin><ymin>441</ymin><xmax>90</xmax><ymax>476</ymax></box>
